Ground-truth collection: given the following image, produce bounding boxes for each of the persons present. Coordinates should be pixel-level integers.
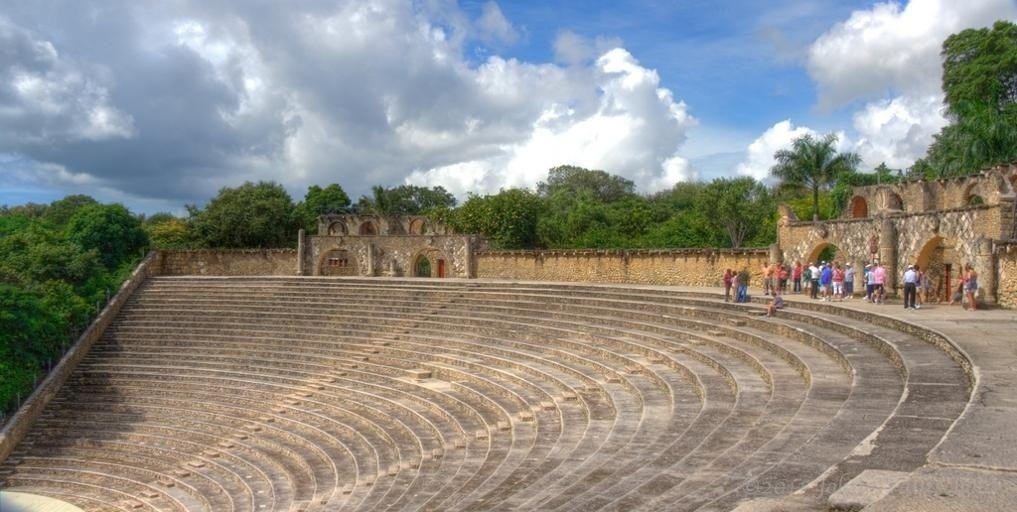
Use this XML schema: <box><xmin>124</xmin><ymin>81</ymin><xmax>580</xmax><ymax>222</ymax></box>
<box><xmin>722</xmin><ymin>259</ymin><xmax>978</xmax><ymax>315</ymax></box>
